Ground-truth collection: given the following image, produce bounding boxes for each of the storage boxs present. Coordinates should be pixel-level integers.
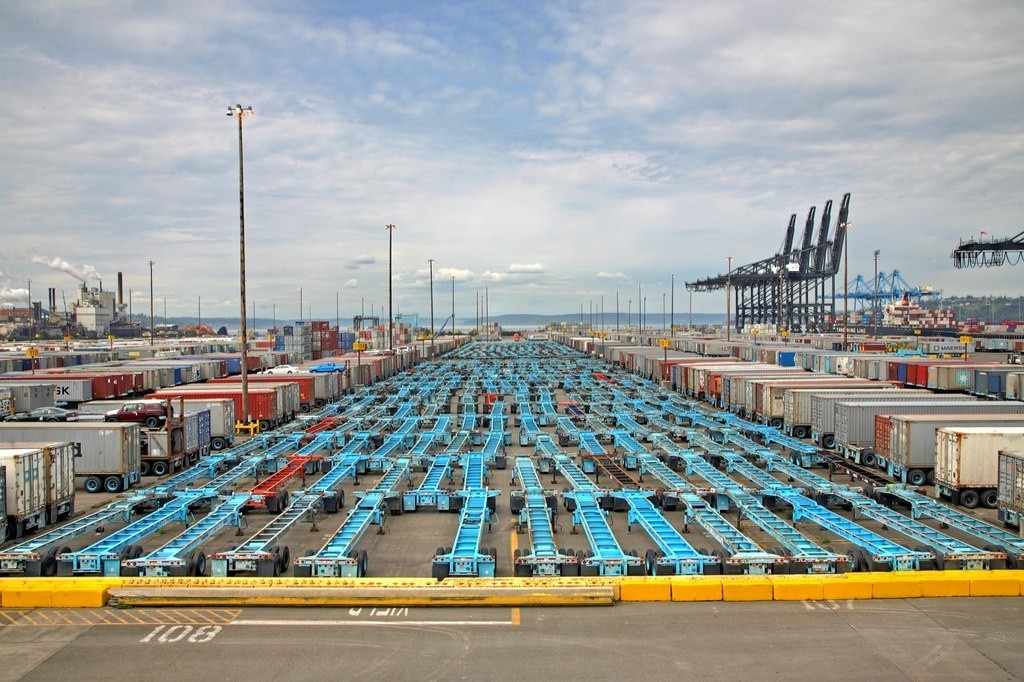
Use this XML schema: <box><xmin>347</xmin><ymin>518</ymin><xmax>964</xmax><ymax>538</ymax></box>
<box><xmin>0</xmin><ymin>312</ymin><xmax>472</xmax><ymax>522</ymax></box>
<box><xmin>574</xmin><ymin>329</ymin><xmax>1024</xmax><ymax>514</ymax></box>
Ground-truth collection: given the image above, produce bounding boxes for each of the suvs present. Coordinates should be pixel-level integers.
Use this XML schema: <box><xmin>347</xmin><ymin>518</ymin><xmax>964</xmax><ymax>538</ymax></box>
<box><xmin>104</xmin><ymin>400</ymin><xmax>174</xmax><ymax>428</ymax></box>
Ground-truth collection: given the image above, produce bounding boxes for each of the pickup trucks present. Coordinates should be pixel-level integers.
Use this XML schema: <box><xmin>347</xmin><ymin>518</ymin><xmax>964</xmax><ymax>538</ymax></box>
<box><xmin>308</xmin><ymin>362</ymin><xmax>345</xmax><ymax>373</ymax></box>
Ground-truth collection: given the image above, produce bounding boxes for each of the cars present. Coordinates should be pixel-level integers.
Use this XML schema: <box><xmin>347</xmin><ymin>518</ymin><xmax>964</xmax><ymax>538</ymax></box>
<box><xmin>4</xmin><ymin>407</ymin><xmax>80</xmax><ymax>423</ymax></box>
<box><xmin>396</xmin><ymin>346</ymin><xmax>409</xmax><ymax>355</ymax></box>
<box><xmin>266</xmin><ymin>364</ymin><xmax>300</xmax><ymax>375</ymax></box>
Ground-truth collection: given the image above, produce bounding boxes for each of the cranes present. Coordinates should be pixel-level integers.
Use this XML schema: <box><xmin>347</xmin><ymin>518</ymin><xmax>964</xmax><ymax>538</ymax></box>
<box><xmin>684</xmin><ymin>192</ymin><xmax>941</xmax><ymax>333</ymax></box>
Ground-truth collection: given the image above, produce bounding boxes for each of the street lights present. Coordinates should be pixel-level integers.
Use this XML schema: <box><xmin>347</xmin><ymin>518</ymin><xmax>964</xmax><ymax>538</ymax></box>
<box><xmin>451</xmin><ymin>276</ymin><xmax>456</xmax><ymax>340</ymax></box>
<box><xmin>637</xmin><ymin>284</ymin><xmax>644</xmax><ymax>334</ymax></box>
<box><xmin>726</xmin><ymin>257</ymin><xmax>734</xmax><ymax>341</ymax></box>
<box><xmin>225</xmin><ymin>105</ymin><xmax>253</xmax><ymax>424</ymax></box>
<box><xmin>644</xmin><ymin>297</ymin><xmax>647</xmax><ymax>331</ymax></box>
<box><xmin>874</xmin><ymin>249</ymin><xmax>881</xmax><ymax>341</ymax></box>
<box><xmin>669</xmin><ymin>275</ymin><xmax>676</xmax><ymax>337</ymax></box>
<box><xmin>663</xmin><ymin>293</ymin><xmax>666</xmax><ymax>332</ymax></box>
<box><xmin>428</xmin><ymin>259</ymin><xmax>435</xmax><ymax>344</ymax></box>
<box><xmin>385</xmin><ymin>224</ymin><xmax>397</xmax><ymax>350</ymax></box>
<box><xmin>629</xmin><ymin>300</ymin><xmax>631</xmax><ymax>328</ymax></box>
